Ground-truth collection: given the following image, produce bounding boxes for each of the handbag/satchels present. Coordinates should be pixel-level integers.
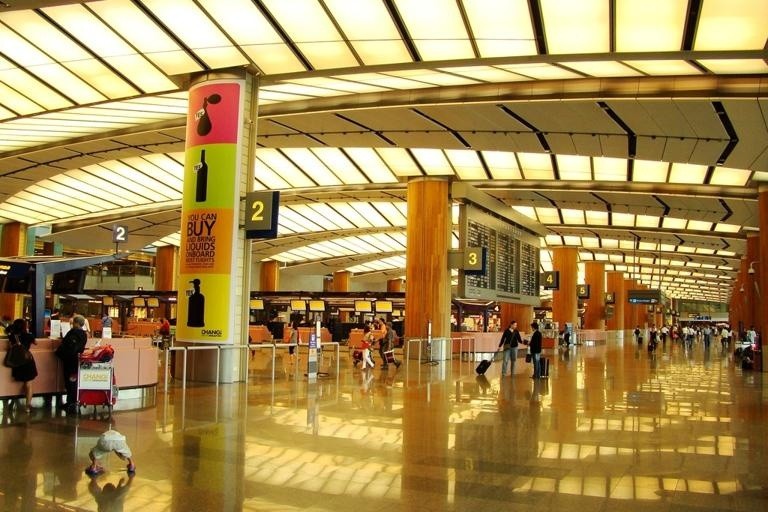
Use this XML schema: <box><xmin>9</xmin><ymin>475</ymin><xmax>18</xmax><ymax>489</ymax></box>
<box><xmin>525</xmin><ymin>354</ymin><xmax>531</xmax><ymax>362</ymax></box>
<box><xmin>383</xmin><ymin>350</ymin><xmax>397</xmax><ymax>364</ymax></box>
<box><xmin>5</xmin><ymin>346</ymin><xmax>26</xmax><ymax>368</ymax></box>
<box><xmin>503</xmin><ymin>344</ymin><xmax>511</xmax><ymax>349</ymax></box>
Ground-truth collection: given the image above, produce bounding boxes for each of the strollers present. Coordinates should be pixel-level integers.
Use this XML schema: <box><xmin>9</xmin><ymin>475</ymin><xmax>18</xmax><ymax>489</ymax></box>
<box><xmin>353</xmin><ymin>339</ymin><xmax>377</xmax><ymax>366</ymax></box>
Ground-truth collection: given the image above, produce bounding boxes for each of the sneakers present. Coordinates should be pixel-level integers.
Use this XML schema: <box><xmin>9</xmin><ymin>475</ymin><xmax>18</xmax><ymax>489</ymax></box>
<box><xmin>89</xmin><ymin>464</ymin><xmax>102</xmax><ymax>473</ymax></box>
<box><xmin>127</xmin><ymin>464</ymin><xmax>135</xmax><ymax>472</ymax></box>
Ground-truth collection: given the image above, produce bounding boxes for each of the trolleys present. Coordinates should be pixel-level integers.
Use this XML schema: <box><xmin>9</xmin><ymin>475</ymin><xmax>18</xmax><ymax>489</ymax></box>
<box><xmin>76</xmin><ymin>353</ymin><xmax>113</xmax><ymax>416</ymax></box>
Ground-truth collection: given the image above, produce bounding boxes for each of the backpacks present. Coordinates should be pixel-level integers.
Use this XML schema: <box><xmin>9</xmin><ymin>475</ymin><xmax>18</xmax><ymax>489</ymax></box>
<box><xmin>635</xmin><ymin>329</ymin><xmax>640</xmax><ymax>334</ymax></box>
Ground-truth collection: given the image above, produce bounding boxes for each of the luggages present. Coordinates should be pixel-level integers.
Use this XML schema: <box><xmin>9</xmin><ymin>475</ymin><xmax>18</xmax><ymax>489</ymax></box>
<box><xmin>648</xmin><ymin>344</ymin><xmax>653</xmax><ymax>351</ymax></box>
<box><xmin>638</xmin><ymin>337</ymin><xmax>642</xmax><ymax>343</ymax></box>
<box><xmin>541</xmin><ymin>358</ymin><xmax>549</xmax><ymax>377</ymax></box>
<box><xmin>476</xmin><ymin>349</ymin><xmax>500</xmax><ymax>375</ymax></box>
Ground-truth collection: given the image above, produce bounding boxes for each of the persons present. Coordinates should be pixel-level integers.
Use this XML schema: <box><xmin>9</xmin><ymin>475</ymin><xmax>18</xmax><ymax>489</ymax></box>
<box><xmin>563</xmin><ymin>324</ymin><xmax>570</xmax><ymax>357</ymax></box>
<box><xmin>6</xmin><ymin>319</ymin><xmax>38</xmax><ymax>413</ymax></box>
<box><xmin>54</xmin><ymin>315</ymin><xmax>87</xmax><ymax>413</ymax></box>
<box><xmin>156</xmin><ymin>319</ymin><xmax>170</xmax><ymax>336</ymax></box>
<box><xmin>526</xmin><ymin>322</ymin><xmax>542</xmax><ymax>379</ymax></box>
<box><xmin>288</xmin><ymin>320</ymin><xmax>301</xmax><ymax>368</ymax></box>
<box><xmin>378</xmin><ymin>318</ymin><xmax>387</xmax><ymax>339</ymax></box>
<box><xmin>376</xmin><ymin>322</ymin><xmax>401</xmax><ymax>369</ymax></box>
<box><xmin>360</xmin><ymin>320</ymin><xmax>375</xmax><ymax>370</ymax></box>
<box><xmin>50</xmin><ymin>309</ymin><xmax>60</xmax><ymax>320</ymax></box>
<box><xmin>101</xmin><ymin>313</ymin><xmax>113</xmax><ymax>339</ymax></box>
<box><xmin>632</xmin><ymin>322</ymin><xmax>759</xmax><ymax>369</ymax></box>
<box><xmin>85</xmin><ymin>429</ymin><xmax>136</xmax><ymax>475</ymax></box>
<box><xmin>497</xmin><ymin>321</ymin><xmax>524</xmax><ymax>378</ymax></box>
<box><xmin>69</xmin><ymin>312</ymin><xmax>90</xmax><ymax>337</ymax></box>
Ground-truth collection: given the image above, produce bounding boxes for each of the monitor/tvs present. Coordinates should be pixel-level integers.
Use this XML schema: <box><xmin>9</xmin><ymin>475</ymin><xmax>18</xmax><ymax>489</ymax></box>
<box><xmin>3</xmin><ymin>263</ymin><xmax>36</xmax><ymax>294</ymax></box>
<box><xmin>51</xmin><ymin>269</ymin><xmax>87</xmax><ymax>295</ymax></box>
<box><xmin>290</xmin><ymin>299</ymin><xmax>307</xmax><ymax>311</ymax></box>
<box><xmin>308</xmin><ymin>299</ymin><xmax>327</xmax><ymax>313</ymax></box>
<box><xmin>131</xmin><ymin>296</ymin><xmax>146</xmax><ymax>307</ymax></box>
<box><xmin>147</xmin><ymin>297</ymin><xmax>160</xmax><ymax>309</ymax></box>
<box><xmin>375</xmin><ymin>300</ymin><xmax>393</xmax><ymax>313</ymax></box>
<box><xmin>249</xmin><ymin>299</ymin><xmax>265</xmax><ymax>310</ymax></box>
<box><xmin>101</xmin><ymin>296</ymin><xmax>115</xmax><ymax>307</ymax></box>
<box><xmin>353</xmin><ymin>300</ymin><xmax>372</xmax><ymax>312</ymax></box>
